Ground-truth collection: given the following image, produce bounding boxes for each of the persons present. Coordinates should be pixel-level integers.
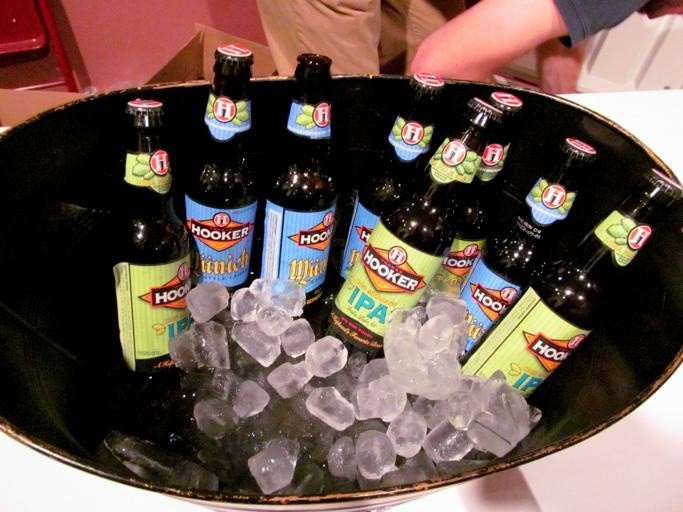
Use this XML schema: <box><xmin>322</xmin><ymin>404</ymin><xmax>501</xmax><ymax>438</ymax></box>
<box><xmin>255</xmin><ymin>1</ymin><xmax>683</xmax><ymax>98</ymax></box>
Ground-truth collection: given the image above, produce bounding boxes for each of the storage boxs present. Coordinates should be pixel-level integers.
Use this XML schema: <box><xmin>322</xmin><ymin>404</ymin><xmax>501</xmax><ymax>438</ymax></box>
<box><xmin>142</xmin><ymin>21</ymin><xmax>277</xmax><ymax>81</ymax></box>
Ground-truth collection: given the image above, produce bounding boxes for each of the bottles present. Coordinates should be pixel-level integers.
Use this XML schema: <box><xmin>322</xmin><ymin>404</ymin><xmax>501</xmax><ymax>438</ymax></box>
<box><xmin>326</xmin><ymin>74</ymin><xmax>446</xmax><ymax>300</ymax></box>
<box><xmin>173</xmin><ymin>43</ymin><xmax>261</xmax><ymax>309</ymax></box>
<box><xmin>459</xmin><ymin>167</ymin><xmax>680</xmax><ymax>401</ymax></box>
<box><xmin>417</xmin><ymin>92</ymin><xmax>523</xmax><ymax>303</ymax></box>
<box><xmin>453</xmin><ymin>138</ymin><xmax>598</xmax><ymax>362</ymax></box>
<box><xmin>249</xmin><ymin>53</ymin><xmax>340</xmax><ymax>316</ymax></box>
<box><xmin>108</xmin><ymin>98</ymin><xmax>192</xmax><ymax>378</ymax></box>
<box><xmin>329</xmin><ymin>97</ymin><xmax>505</xmax><ymax>364</ymax></box>
